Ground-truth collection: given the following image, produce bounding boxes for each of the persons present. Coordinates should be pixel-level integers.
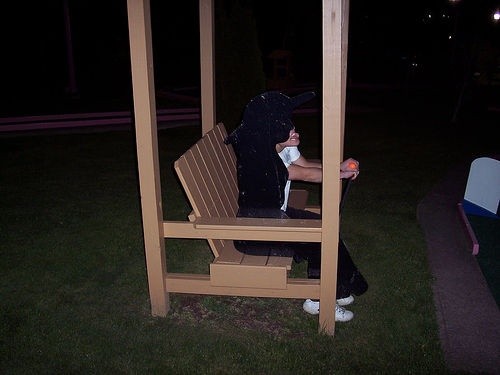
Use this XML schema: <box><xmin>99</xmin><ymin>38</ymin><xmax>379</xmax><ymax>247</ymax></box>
<box><xmin>276</xmin><ymin>125</ymin><xmax>360</xmax><ymax>322</ymax></box>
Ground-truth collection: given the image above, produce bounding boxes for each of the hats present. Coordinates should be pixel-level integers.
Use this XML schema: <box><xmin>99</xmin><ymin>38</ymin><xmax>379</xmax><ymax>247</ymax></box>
<box><xmin>223</xmin><ymin>91</ymin><xmax>315</xmax><ymax>144</ymax></box>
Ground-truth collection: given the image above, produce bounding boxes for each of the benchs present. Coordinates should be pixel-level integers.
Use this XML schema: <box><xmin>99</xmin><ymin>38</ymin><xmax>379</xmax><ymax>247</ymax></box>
<box><xmin>173</xmin><ymin>123</ymin><xmax>310</xmax><ymax>288</ymax></box>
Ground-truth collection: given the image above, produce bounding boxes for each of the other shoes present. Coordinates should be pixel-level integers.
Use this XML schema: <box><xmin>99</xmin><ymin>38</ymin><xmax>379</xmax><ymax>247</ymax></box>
<box><xmin>303</xmin><ymin>299</ymin><xmax>354</xmax><ymax>322</ymax></box>
<box><xmin>336</xmin><ymin>295</ymin><xmax>354</xmax><ymax>305</ymax></box>
